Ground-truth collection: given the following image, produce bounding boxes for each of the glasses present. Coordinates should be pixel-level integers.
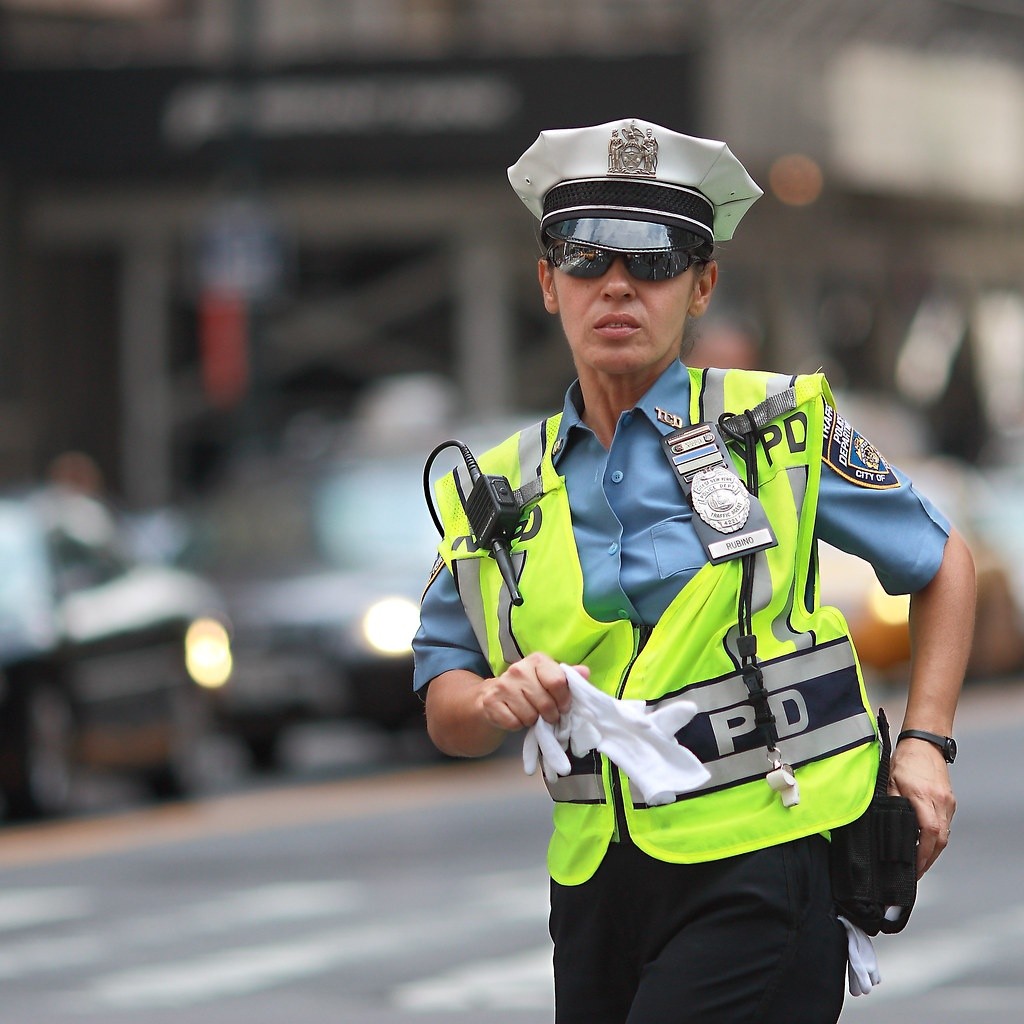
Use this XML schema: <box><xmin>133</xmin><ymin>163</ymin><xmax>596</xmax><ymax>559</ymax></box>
<box><xmin>547</xmin><ymin>240</ymin><xmax>710</xmax><ymax>280</ymax></box>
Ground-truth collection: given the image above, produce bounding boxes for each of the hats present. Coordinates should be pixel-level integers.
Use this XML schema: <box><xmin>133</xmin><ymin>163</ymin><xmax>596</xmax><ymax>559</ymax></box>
<box><xmin>507</xmin><ymin>118</ymin><xmax>766</xmax><ymax>252</ymax></box>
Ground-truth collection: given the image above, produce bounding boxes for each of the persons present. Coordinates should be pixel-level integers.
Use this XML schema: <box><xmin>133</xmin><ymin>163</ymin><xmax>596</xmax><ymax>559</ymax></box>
<box><xmin>412</xmin><ymin>112</ymin><xmax>984</xmax><ymax>1024</ymax></box>
<box><xmin>318</xmin><ymin>369</ymin><xmax>496</xmax><ymax>580</ymax></box>
<box><xmin>0</xmin><ymin>442</ymin><xmax>124</xmax><ymax>823</ymax></box>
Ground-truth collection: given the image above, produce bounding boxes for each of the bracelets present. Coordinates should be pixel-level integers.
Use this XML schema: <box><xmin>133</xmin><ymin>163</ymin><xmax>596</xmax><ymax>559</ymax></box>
<box><xmin>894</xmin><ymin>729</ymin><xmax>959</xmax><ymax>766</ymax></box>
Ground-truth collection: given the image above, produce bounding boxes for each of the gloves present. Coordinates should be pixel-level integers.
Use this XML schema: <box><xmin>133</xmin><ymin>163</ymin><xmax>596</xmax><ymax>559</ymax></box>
<box><xmin>522</xmin><ymin>664</ymin><xmax>713</xmax><ymax>805</ymax></box>
<box><xmin>836</xmin><ymin>916</ymin><xmax>882</xmax><ymax>997</ymax></box>
